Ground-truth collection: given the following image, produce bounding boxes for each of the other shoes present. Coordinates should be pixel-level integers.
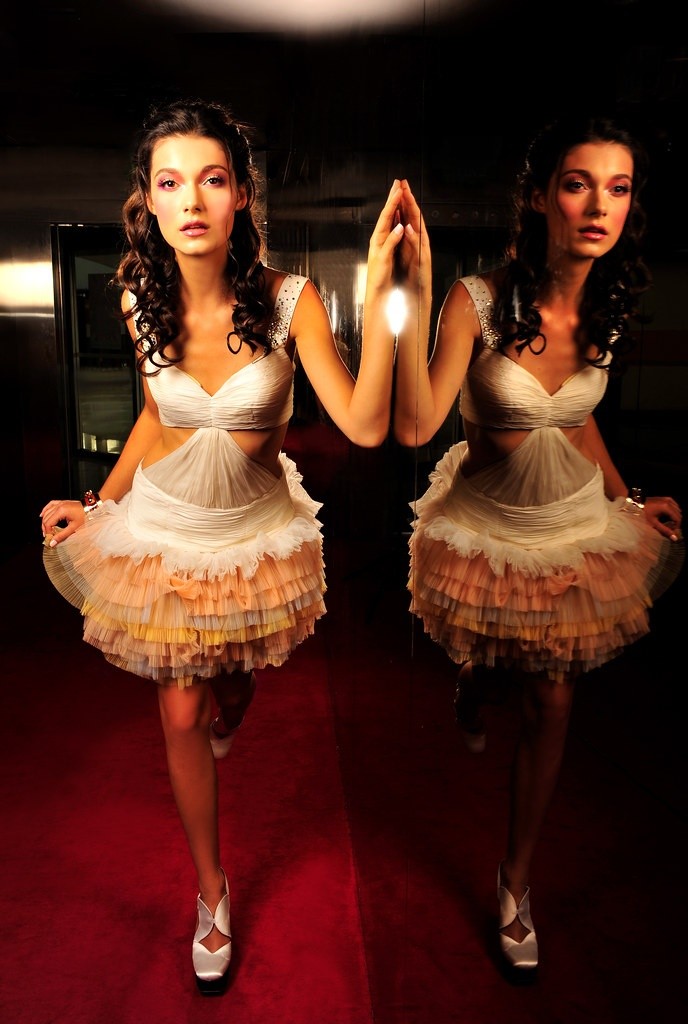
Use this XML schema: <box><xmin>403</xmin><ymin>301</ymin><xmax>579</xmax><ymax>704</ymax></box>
<box><xmin>458</xmin><ymin>720</ymin><xmax>492</xmax><ymax>753</ymax></box>
<box><xmin>209</xmin><ymin>712</ymin><xmax>245</xmax><ymax>758</ymax></box>
<box><xmin>496</xmin><ymin>863</ymin><xmax>538</xmax><ymax>968</ymax></box>
<box><xmin>190</xmin><ymin>867</ymin><xmax>234</xmax><ymax>986</ymax></box>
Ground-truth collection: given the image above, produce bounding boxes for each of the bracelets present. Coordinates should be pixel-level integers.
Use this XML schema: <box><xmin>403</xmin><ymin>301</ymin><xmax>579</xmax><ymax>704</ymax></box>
<box><xmin>624</xmin><ymin>487</ymin><xmax>646</xmax><ymax>517</ymax></box>
<box><xmin>79</xmin><ymin>490</ymin><xmax>105</xmax><ymax>519</ymax></box>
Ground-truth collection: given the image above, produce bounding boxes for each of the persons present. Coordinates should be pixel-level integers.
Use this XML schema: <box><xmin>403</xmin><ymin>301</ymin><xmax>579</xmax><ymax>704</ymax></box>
<box><xmin>399</xmin><ymin>120</ymin><xmax>683</xmax><ymax>974</ymax></box>
<box><xmin>40</xmin><ymin>100</ymin><xmax>405</xmax><ymax>988</ymax></box>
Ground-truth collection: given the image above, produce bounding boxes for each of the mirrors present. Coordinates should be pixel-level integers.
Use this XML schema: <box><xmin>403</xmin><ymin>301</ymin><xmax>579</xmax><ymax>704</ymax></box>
<box><xmin>282</xmin><ymin>0</ymin><xmax>688</xmax><ymax>1024</ymax></box>
